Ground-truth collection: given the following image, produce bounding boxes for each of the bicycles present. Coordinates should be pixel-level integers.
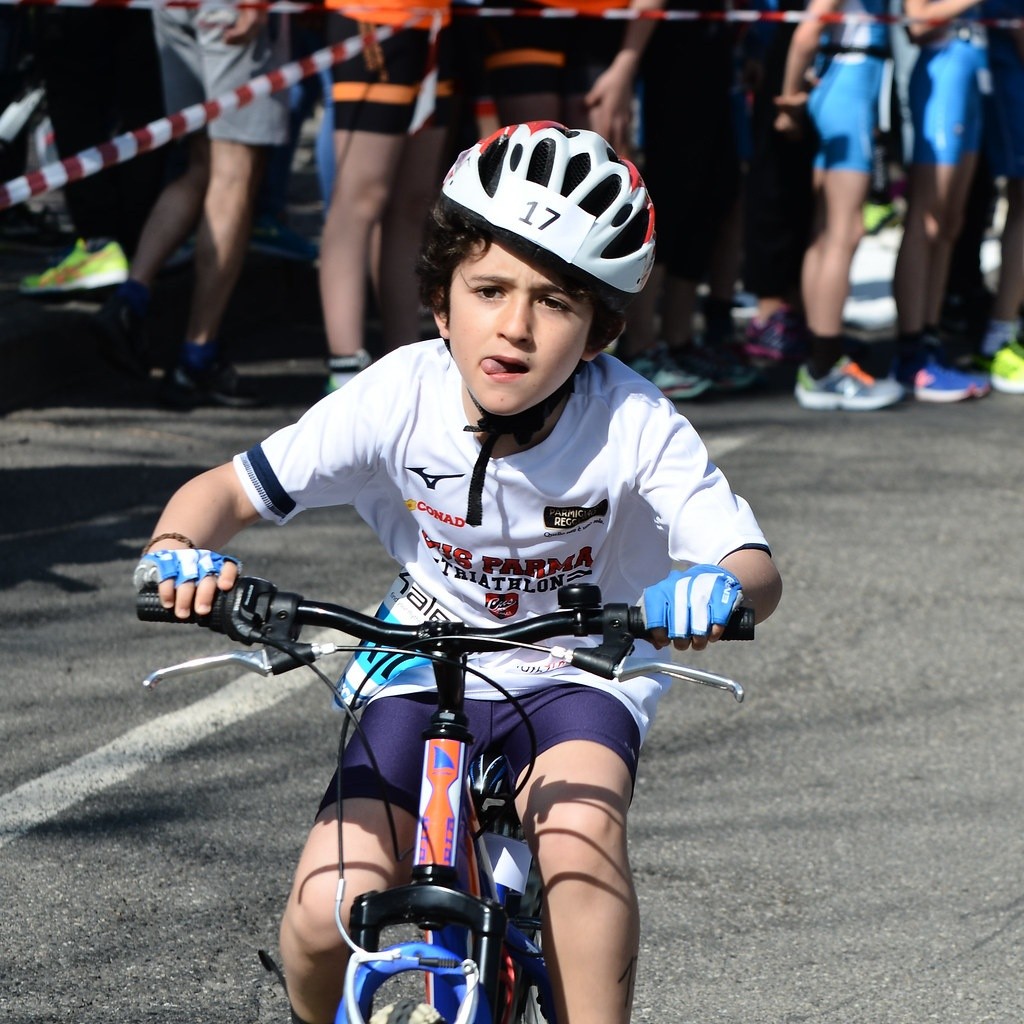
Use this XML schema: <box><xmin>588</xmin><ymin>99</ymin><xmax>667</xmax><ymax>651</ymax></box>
<box><xmin>136</xmin><ymin>573</ymin><xmax>759</xmax><ymax>1023</ymax></box>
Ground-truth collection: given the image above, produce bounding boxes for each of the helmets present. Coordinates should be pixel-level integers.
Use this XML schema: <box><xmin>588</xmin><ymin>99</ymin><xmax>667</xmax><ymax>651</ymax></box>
<box><xmin>441</xmin><ymin>120</ymin><xmax>655</xmax><ymax>305</ymax></box>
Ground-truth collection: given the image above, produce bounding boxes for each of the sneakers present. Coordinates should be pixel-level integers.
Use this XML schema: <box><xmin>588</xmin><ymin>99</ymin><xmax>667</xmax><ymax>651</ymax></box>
<box><xmin>973</xmin><ymin>342</ymin><xmax>1024</xmax><ymax>393</ymax></box>
<box><xmin>93</xmin><ymin>301</ymin><xmax>158</xmax><ymax>379</ymax></box>
<box><xmin>20</xmin><ymin>240</ymin><xmax>128</xmax><ymax>294</ymax></box>
<box><xmin>621</xmin><ymin>358</ymin><xmax>710</xmax><ymax>398</ymax></box>
<box><xmin>800</xmin><ymin>359</ymin><xmax>901</xmax><ymax>411</ymax></box>
<box><xmin>892</xmin><ymin>356</ymin><xmax>991</xmax><ymax>402</ymax></box>
<box><xmin>161</xmin><ymin>365</ymin><xmax>274</xmax><ymax>406</ymax></box>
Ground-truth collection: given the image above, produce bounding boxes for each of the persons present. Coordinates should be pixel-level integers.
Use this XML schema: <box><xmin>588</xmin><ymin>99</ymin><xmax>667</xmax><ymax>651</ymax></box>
<box><xmin>732</xmin><ymin>0</ymin><xmax>820</xmax><ymax>362</ymax></box>
<box><xmin>634</xmin><ymin>0</ymin><xmax>763</xmax><ymax>393</ymax></box>
<box><xmin>774</xmin><ymin>0</ymin><xmax>909</xmax><ymax>412</ymax></box>
<box><xmin>133</xmin><ymin>118</ymin><xmax>782</xmax><ymax>1022</ymax></box>
<box><xmin>887</xmin><ymin>0</ymin><xmax>995</xmax><ymax>407</ymax></box>
<box><xmin>318</xmin><ymin>0</ymin><xmax>459</xmax><ymax>399</ymax></box>
<box><xmin>12</xmin><ymin>1</ymin><xmax>138</xmax><ymax>292</ymax></box>
<box><xmin>117</xmin><ymin>1</ymin><xmax>303</xmax><ymax>409</ymax></box>
<box><xmin>448</xmin><ymin>0</ymin><xmax>709</xmax><ymax>403</ymax></box>
<box><xmin>976</xmin><ymin>0</ymin><xmax>1023</xmax><ymax>395</ymax></box>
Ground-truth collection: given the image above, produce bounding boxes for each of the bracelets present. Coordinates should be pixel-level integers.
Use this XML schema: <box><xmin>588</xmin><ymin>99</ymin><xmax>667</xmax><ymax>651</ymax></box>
<box><xmin>141</xmin><ymin>532</ymin><xmax>197</xmax><ymax>558</ymax></box>
